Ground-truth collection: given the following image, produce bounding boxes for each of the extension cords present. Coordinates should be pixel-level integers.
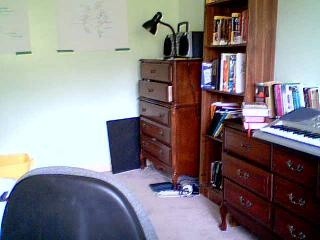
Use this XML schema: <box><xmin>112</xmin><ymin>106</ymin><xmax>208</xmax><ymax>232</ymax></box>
<box><xmin>157</xmin><ymin>190</ymin><xmax>186</xmax><ymax>199</ymax></box>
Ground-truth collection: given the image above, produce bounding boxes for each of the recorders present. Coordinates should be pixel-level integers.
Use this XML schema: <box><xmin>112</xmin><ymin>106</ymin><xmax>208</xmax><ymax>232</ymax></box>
<box><xmin>163</xmin><ymin>21</ymin><xmax>203</xmax><ymax>58</ymax></box>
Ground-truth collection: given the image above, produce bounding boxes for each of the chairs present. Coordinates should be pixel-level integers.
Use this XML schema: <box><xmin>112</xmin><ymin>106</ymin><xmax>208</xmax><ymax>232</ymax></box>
<box><xmin>0</xmin><ymin>166</ymin><xmax>157</xmax><ymax>240</ymax></box>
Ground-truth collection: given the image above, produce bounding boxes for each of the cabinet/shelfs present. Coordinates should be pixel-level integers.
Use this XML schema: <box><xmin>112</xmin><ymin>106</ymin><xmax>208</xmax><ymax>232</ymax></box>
<box><xmin>138</xmin><ymin>58</ymin><xmax>202</xmax><ymax>191</ymax></box>
<box><xmin>198</xmin><ymin>0</ymin><xmax>279</xmax><ymax>206</ymax></box>
<box><xmin>217</xmin><ymin>117</ymin><xmax>320</xmax><ymax>240</ymax></box>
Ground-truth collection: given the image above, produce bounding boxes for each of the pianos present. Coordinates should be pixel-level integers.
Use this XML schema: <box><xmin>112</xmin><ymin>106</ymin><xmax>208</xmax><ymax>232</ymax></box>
<box><xmin>252</xmin><ymin>107</ymin><xmax>320</xmax><ymax>157</ymax></box>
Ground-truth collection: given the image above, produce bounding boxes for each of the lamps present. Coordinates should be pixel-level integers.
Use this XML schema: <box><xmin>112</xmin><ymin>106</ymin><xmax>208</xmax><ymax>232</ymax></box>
<box><xmin>143</xmin><ymin>12</ymin><xmax>188</xmax><ymax>60</ymax></box>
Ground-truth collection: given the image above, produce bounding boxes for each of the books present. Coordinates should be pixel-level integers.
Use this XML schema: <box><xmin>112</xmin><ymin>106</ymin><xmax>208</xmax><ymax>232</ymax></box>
<box><xmin>256</xmin><ymin>81</ymin><xmax>320</xmax><ymax>118</ymax></box>
<box><xmin>206</xmin><ymin>101</ymin><xmax>242</xmax><ymax>137</ymax></box>
<box><xmin>242</xmin><ymin>101</ymin><xmax>271</xmax><ymax>131</ymax></box>
<box><xmin>211</xmin><ymin>161</ymin><xmax>223</xmax><ymax>190</ymax></box>
<box><xmin>201</xmin><ymin>52</ymin><xmax>246</xmax><ymax>95</ymax></box>
<box><xmin>213</xmin><ymin>10</ymin><xmax>249</xmax><ymax>45</ymax></box>
